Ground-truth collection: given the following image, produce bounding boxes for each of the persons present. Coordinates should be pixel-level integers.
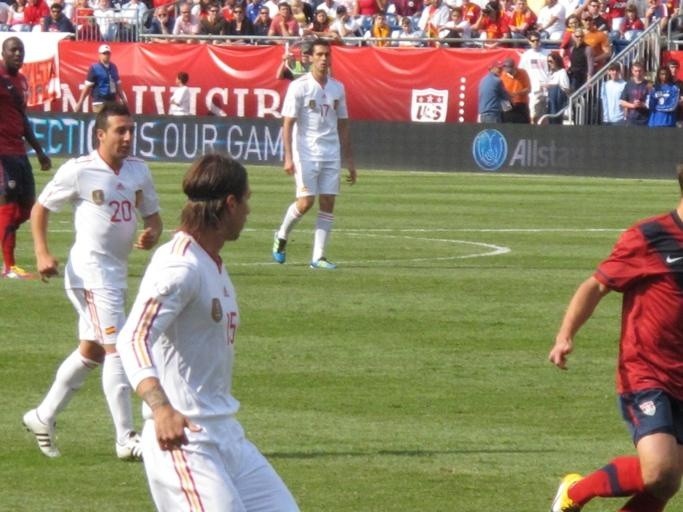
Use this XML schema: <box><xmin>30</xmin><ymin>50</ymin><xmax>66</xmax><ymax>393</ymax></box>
<box><xmin>477</xmin><ymin>14</ymin><xmax>678</xmax><ymax>124</ymax></box>
<box><xmin>110</xmin><ymin>153</ymin><xmax>300</xmax><ymax>512</ymax></box>
<box><xmin>266</xmin><ymin>37</ymin><xmax>357</xmax><ymax>270</ymax></box>
<box><xmin>18</xmin><ymin>99</ymin><xmax>164</xmax><ymax>459</ymax></box>
<box><xmin>75</xmin><ymin>44</ymin><xmax>128</xmax><ymax>113</ymax></box>
<box><xmin>544</xmin><ymin>162</ymin><xmax>681</xmax><ymax>511</ymax></box>
<box><xmin>276</xmin><ymin>40</ymin><xmax>332</xmax><ymax>81</ymax></box>
<box><xmin>2</xmin><ymin>2</ymin><xmax>678</xmax><ymax>47</ymax></box>
<box><xmin>167</xmin><ymin>72</ymin><xmax>194</xmax><ymax>115</ymax></box>
<box><xmin>0</xmin><ymin>35</ymin><xmax>50</xmax><ymax>282</ymax></box>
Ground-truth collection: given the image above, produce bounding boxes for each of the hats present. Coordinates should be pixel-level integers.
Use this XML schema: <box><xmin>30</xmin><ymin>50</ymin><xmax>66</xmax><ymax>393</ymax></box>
<box><xmin>609</xmin><ymin>63</ymin><xmax>620</xmax><ymax>70</ymax></box>
<box><xmin>99</xmin><ymin>45</ymin><xmax>111</xmax><ymax>53</ymax></box>
<box><xmin>482</xmin><ymin>3</ymin><xmax>498</xmax><ymax>12</ymax></box>
<box><xmin>301</xmin><ymin>43</ymin><xmax>312</xmax><ymax>53</ymax></box>
<box><xmin>488</xmin><ymin>59</ymin><xmax>505</xmax><ymax>70</ymax></box>
<box><xmin>336</xmin><ymin>6</ymin><xmax>346</xmax><ymax>12</ymax></box>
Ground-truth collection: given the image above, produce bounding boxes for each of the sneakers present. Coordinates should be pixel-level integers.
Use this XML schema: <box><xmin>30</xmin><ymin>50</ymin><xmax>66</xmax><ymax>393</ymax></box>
<box><xmin>115</xmin><ymin>431</ymin><xmax>143</xmax><ymax>461</ymax></box>
<box><xmin>272</xmin><ymin>233</ymin><xmax>287</xmax><ymax>262</ymax></box>
<box><xmin>310</xmin><ymin>257</ymin><xmax>336</xmax><ymax>269</ymax></box>
<box><xmin>23</xmin><ymin>409</ymin><xmax>60</xmax><ymax>459</ymax></box>
<box><xmin>1</xmin><ymin>263</ymin><xmax>35</xmax><ymax>279</ymax></box>
<box><xmin>552</xmin><ymin>473</ymin><xmax>584</xmax><ymax>512</ymax></box>
